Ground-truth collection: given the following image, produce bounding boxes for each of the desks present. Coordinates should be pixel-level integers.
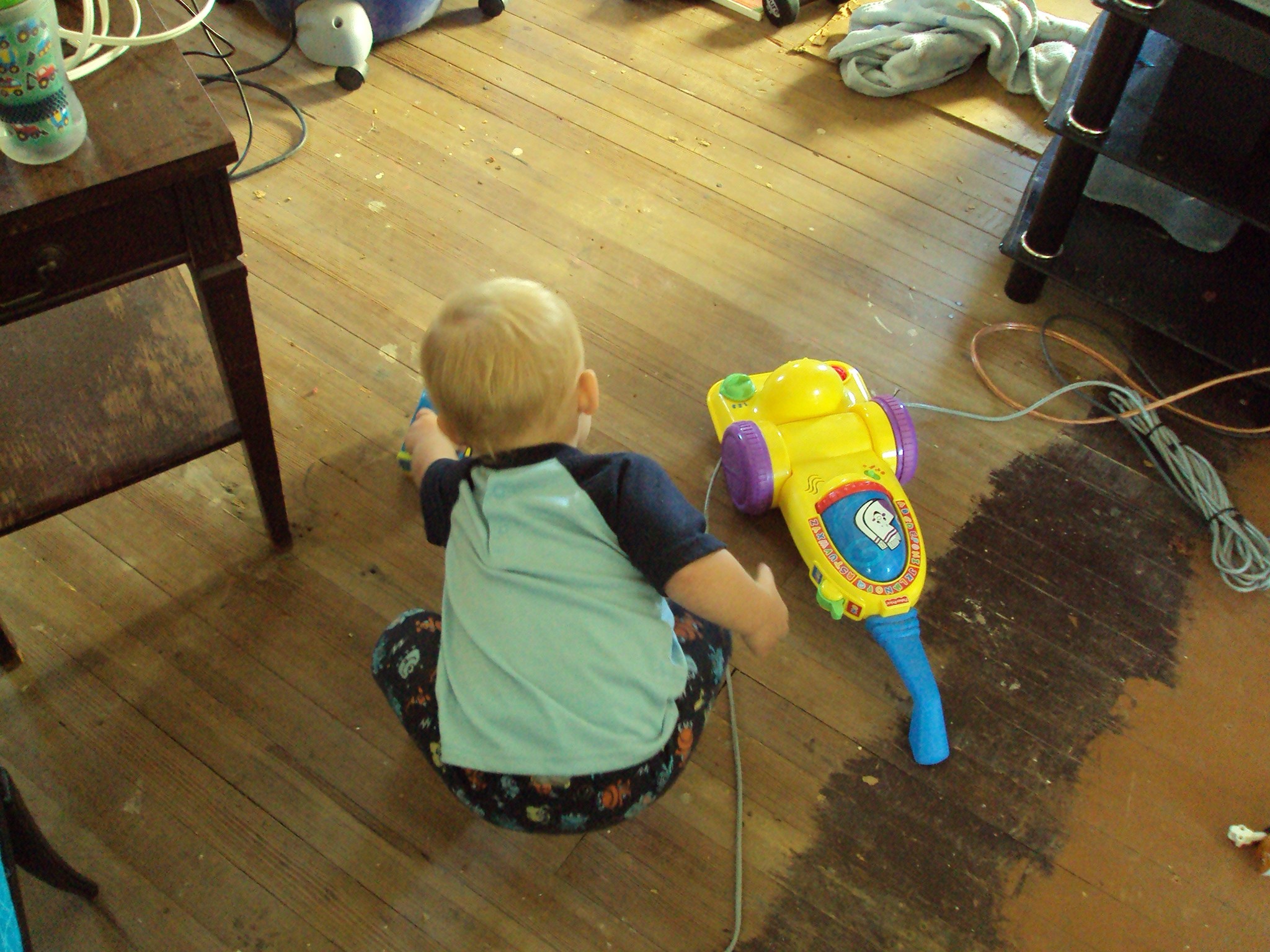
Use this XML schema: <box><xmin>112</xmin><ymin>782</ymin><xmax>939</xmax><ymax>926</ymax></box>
<box><xmin>3</xmin><ymin>0</ymin><xmax>296</xmax><ymax>677</ymax></box>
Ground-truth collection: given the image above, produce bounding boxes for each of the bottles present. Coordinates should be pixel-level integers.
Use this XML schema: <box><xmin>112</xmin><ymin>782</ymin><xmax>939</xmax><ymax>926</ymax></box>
<box><xmin>1</xmin><ymin>1</ymin><xmax>86</xmax><ymax>166</ymax></box>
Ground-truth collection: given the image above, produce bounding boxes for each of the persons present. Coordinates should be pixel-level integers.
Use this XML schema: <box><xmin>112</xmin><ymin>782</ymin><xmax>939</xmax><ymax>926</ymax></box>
<box><xmin>370</xmin><ymin>275</ymin><xmax>790</xmax><ymax>836</ymax></box>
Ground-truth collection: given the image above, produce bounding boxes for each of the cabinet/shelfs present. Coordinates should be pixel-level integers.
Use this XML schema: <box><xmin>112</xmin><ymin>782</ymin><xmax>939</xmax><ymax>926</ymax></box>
<box><xmin>998</xmin><ymin>0</ymin><xmax>1270</xmax><ymax>408</ymax></box>
